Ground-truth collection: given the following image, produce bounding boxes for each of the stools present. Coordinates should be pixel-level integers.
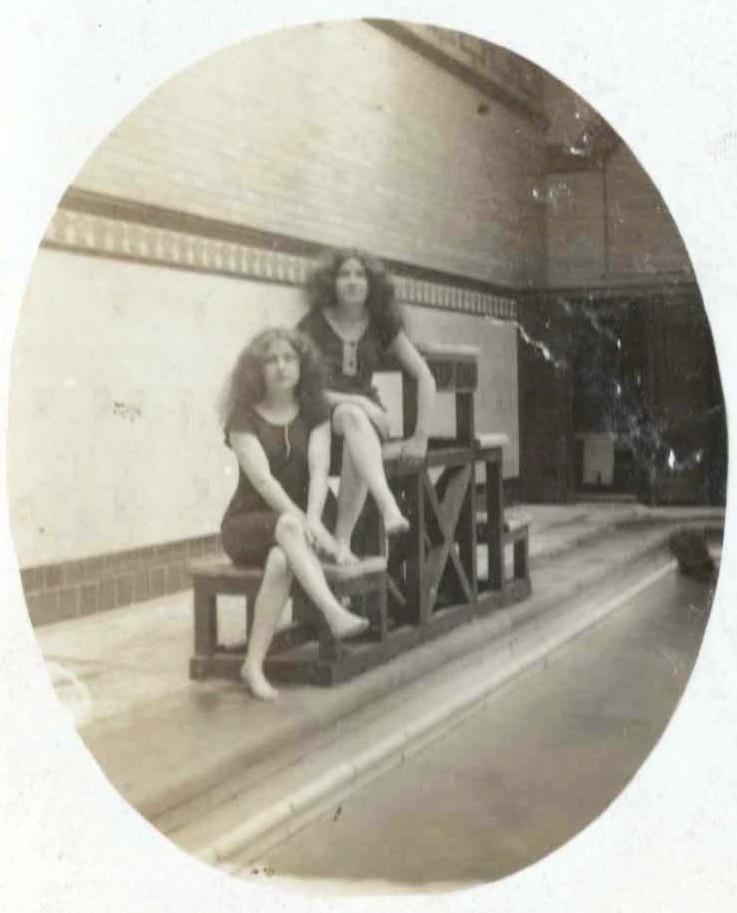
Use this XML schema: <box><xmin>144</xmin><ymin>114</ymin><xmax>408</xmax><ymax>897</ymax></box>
<box><xmin>177</xmin><ymin>347</ymin><xmax>531</xmax><ymax>688</ymax></box>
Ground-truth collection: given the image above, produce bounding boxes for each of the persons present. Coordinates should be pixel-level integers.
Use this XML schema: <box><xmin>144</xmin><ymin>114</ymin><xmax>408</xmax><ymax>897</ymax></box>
<box><xmin>295</xmin><ymin>248</ymin><xmax>441</xmax><ymax>566</ymax></box>
<box><xmin>218</xmin><ymin>326</ymin><xmax>369</xmax><ymax>702</ymax></box>
